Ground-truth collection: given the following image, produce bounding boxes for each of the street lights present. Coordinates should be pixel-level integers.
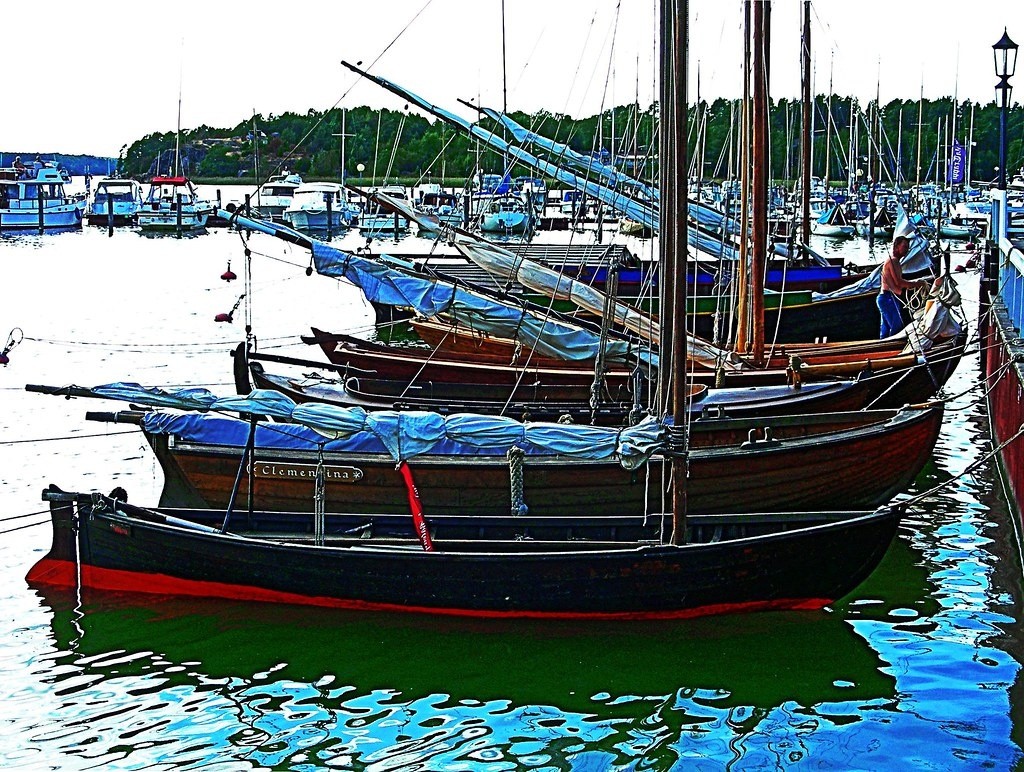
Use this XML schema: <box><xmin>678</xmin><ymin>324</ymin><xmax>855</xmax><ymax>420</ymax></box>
<box><xmin>357</xmin><ymin>164</ymin><xmax>365</xmax><ymax>178</ymax></box>
<box><xmin>992</xmin><ymin>26</ymin><xmax>1020</xmax><ymax>193</ymax></box>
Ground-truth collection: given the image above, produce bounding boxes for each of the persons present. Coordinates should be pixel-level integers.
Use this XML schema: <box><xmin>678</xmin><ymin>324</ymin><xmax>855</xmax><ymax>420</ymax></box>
<box><xmin>866</xmin><ymin>176</ymin><xmax>874</xmax><ymax>191</ymax></box>
<box><xmin>852</xmin><ymin>181</ymin><xmax>858</xmax><ymax>193</ymax></box>
<box><xmin>876</xmin><ymin>236</ymin><xmax>927</xmax><ymax>339</ymax></box>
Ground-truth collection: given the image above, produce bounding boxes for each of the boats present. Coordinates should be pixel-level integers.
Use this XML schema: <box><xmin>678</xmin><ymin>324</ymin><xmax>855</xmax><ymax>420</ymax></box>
<box><xmin>0</xmin><ymin>161</ymin><xmax>87</xmax><ymax>227</ymax></box>
<box><xmin>411</xmin><ymin>183</ymin><xmax>463</xmax><ymax>231</ymax></box>
<box><xmin>86</xmin><ymin>179</ymin><xmax>144</xmax><ymax>223</ymax></box>
<box><xmin>458</xmin><ymin>0</ymin><xmax>1024</xmax><ymax>238</ymax></box>
<box><xmin>284</xmin><ymin>182</ymin><xmax>352</xmax><ymax>231</ymax></box>
<box><xmin>357</xmin><ymin>186</ymin><xmax>410</xmax><ymax>232</ymax></box>
<box><xmin>253</xmin><ymin>175</ymin><xmax>304</xmax><ymax>215</ymax></box>
<box><xmin>137</xmin><ymin>70</ymin><xmax>213</xmax><ymax>231</ymax></box>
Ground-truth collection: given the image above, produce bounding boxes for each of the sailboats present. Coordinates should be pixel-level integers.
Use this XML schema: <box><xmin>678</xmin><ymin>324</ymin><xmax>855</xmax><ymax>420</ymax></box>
<box><xmin>24</xmin><ymin>0</ymin><xmax>908</xmax><ymax>633</ymax></box>
<box><xmin>184</xmin><ymin>0</ymin><xmax>969</xmax><ymax>417</ymax></box>
<box><xmin>87</xmin><ymin>0</ymin><xmax>945</xmax><ymax>510</ymax></box>
